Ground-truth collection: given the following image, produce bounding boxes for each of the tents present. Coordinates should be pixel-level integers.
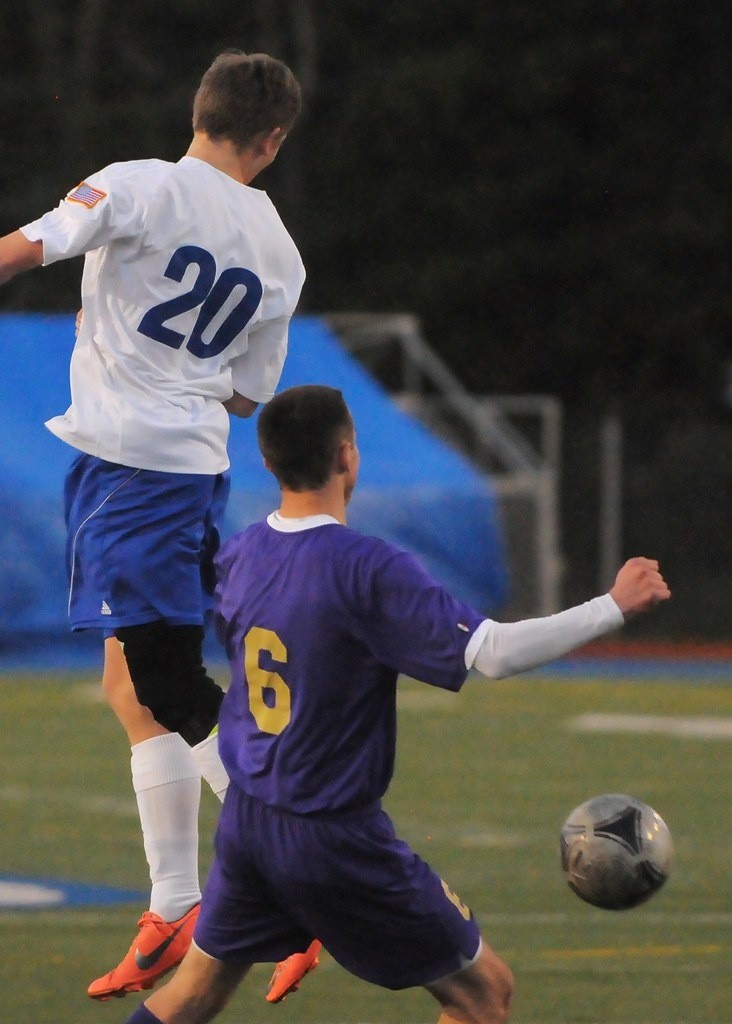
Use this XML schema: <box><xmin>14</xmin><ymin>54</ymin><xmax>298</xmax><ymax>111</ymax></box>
<box><xmin>0</xmin><ymin>311</ymin><xmax>509</xmax><ymax>679</ymax></box>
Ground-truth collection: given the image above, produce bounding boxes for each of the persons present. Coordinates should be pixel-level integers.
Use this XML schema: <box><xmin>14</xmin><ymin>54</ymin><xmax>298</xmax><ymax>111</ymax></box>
<box><xmin>125</xmin><ymin>388</ymin><xmax>672</xmax><ymax>1024</ymax></box>
<box><xmin>0</xmin><ymin>52</ymin><xmax>323</xmax><ymax>1005</ymax></box>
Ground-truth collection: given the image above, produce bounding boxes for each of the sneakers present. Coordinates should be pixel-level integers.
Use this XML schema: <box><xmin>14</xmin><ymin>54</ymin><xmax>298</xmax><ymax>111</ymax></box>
<box><xmin>87</xmin><ymin>901</ymin><xmax>201</xmax><ymax>1001</ymax></box>
<box><xmin>265</xmin><ymin>939</ymin><xmax>324</xmax><ymax>1005</ymax></box>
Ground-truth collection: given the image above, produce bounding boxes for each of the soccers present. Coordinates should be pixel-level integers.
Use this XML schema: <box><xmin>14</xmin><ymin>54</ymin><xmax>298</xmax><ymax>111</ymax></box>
<box><xmin>560</xmin><ymin>791</ymin><xmax>674</xmax><ymax>911</ymax></box>
